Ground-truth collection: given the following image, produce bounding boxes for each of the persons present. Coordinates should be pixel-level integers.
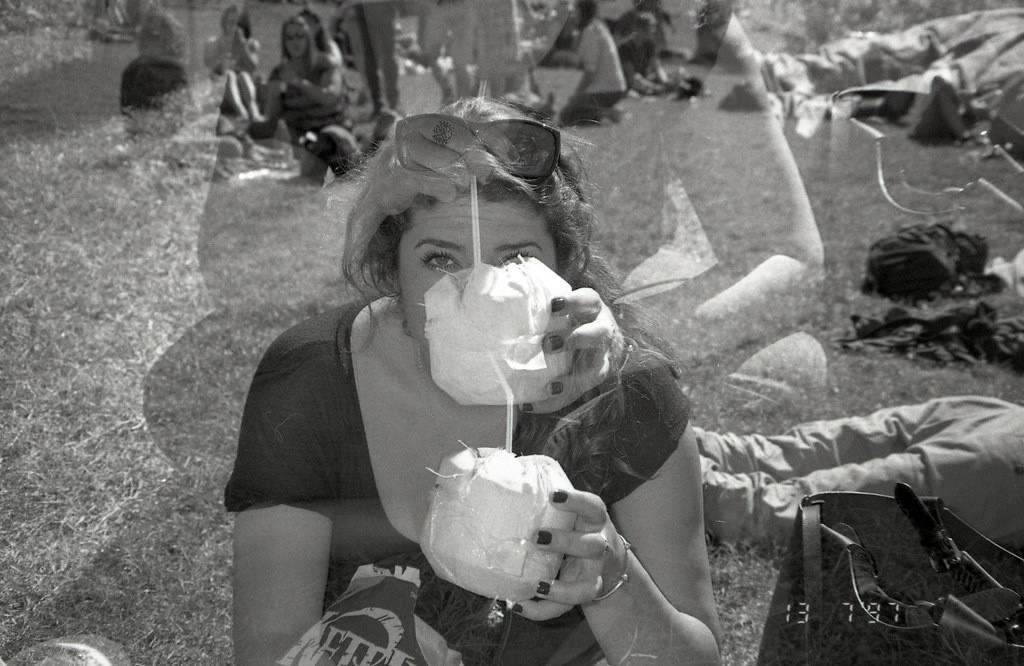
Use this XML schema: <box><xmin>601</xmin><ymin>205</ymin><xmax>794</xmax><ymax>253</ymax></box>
<box><xmin>153</xmin><ymin>0</ymin><xmax>827</xmax><ymax>487</ymax></box>
<box><xmin>755</xmin><ymin>6</ymin><xmax>1024</xmax><ymax>145</ymax></box>
<box><xmin>224</xmin><ymin>100</ymin><xmax>722</xmax><ymax>664</ymax></box>
<box><xmin>95</xmin><ymin>0</ymin><xmax>680</xmax><ymax>185</ymax></box>
<box><xmin>560</xmin><ymin>0</ymin><xmax>632</xmax><ymax>124</ymax></box>
<box><xmin>696</xmin><ymin>395</ymin><xmax>1024</xmax><ymax>556</ymax></box>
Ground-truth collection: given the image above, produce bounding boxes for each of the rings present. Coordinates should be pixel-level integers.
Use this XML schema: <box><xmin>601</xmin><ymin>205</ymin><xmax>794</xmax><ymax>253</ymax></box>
<box><xmin>600</xmin><ymin>534</ymin><xmax>609</xmax><ymax>559</ymax></box>
<box><xmin>432</xmin><ymin>120</ymin><xmax>453</xmax><ymax>143</ymax></box>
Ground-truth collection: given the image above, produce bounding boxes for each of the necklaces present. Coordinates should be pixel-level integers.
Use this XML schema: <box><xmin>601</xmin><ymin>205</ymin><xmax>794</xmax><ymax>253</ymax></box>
<box><xmin>418</xmin><ymin>345</ymin><xmax>439</xmax><ymax>437</ymax></box>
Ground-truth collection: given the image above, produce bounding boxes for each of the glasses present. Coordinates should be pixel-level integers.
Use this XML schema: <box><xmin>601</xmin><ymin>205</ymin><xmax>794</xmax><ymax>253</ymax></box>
<box><xmin>394</xmin><ymin>113</ymin><xmax>561</xmax><ymax>179</ymax></box>
<box><xmin>286</xmin><ymin>32</ymin><xmax>306</xmax><ymax>40</ymax></box>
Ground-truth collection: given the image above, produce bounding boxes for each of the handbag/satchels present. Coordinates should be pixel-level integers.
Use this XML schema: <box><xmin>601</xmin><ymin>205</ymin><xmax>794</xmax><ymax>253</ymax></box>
<box><xmin>758</xmin><ymin>482</ymin><xmax>1024</xmax><ymax>666</ymax></box>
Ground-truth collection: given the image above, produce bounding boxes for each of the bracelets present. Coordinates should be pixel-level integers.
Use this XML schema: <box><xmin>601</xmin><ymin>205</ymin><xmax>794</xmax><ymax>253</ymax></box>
<box><xmin>593</xmin><ymin>534</ymin><xmax>630</xmax><ymax>601</ymax></box>
